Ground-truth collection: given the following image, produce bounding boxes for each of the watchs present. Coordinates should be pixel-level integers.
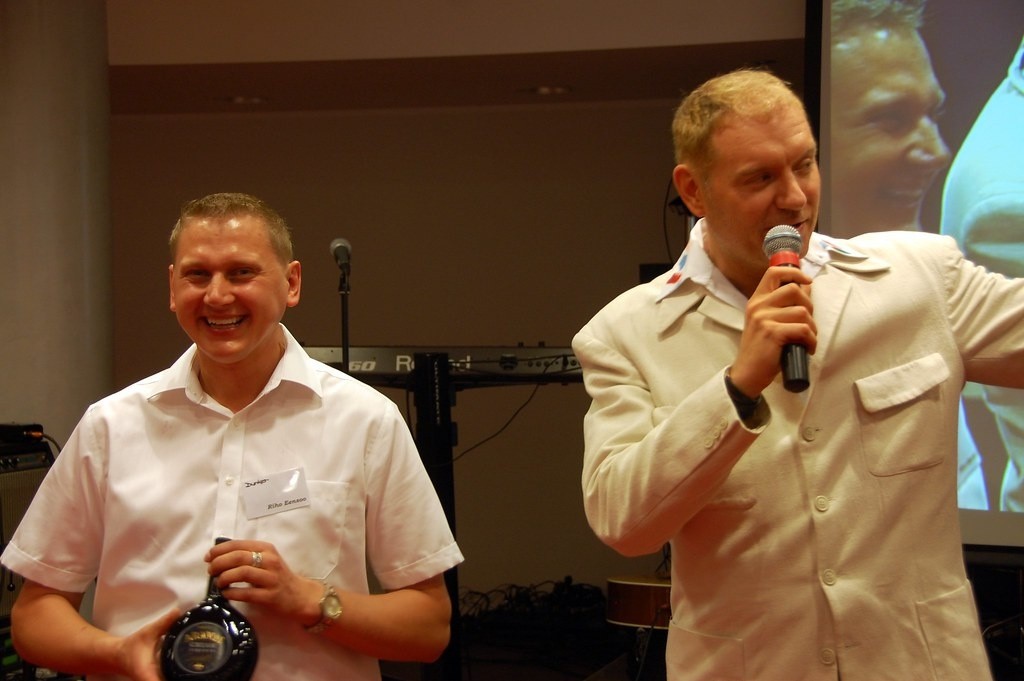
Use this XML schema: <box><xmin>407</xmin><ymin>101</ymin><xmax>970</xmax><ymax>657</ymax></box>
<box><xmin>306</xmin><ymin>581</ymin><xmax>343</xmax><ymax>633</ymax></box>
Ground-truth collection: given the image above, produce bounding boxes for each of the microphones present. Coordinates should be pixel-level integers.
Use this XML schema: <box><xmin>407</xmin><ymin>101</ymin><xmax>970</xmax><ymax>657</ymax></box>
<box><xmin>330</xmin><ymin>237</ymin><xmax>353</xmax><ymax>276</ymax></box>
<box><xmin>762</xmin><ymin>224</ymin><xmax>810</xmax><ymax>393</ymax></box>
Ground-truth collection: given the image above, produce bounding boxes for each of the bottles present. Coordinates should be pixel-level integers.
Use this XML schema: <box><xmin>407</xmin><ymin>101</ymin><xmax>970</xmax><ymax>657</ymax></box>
<box><xmin>160</xmin><ymin>538</ymin><xmax>258</xmax><ymax>681</ymax></box>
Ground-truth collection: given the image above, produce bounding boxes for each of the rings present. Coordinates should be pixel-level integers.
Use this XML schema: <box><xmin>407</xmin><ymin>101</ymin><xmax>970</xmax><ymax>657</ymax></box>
<box><xmin>253</xmin><ymin>552</ymin><xmax>261</xmax><ymax>567</ymax></box>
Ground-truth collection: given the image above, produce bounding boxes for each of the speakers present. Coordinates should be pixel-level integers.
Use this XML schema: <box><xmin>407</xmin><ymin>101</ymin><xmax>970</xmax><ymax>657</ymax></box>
<box><xmin>0</xmin><ymin>440</ymin><xmax>55</xmax><ymax>627</ymax></box>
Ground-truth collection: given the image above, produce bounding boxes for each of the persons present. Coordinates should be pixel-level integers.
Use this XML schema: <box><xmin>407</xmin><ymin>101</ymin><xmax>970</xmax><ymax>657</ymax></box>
<box><xmin>572</xmin><ymin>71</ymin><xmax>1024</xmax><ymax>681</ymax></box>
<box><xmin>0</xmin><ymin>192</ymin><xmax>465</xmax><ymax>681</ymax></box>
<box><xmin>941</xmin><ymin>40</ymin><xmax>1024</xmax><ymax>511</ymax></box>
<box><xmin>830</xmin><ymin>0</ymin><xmax>990</xmax><ymax>511</ymax></box>
